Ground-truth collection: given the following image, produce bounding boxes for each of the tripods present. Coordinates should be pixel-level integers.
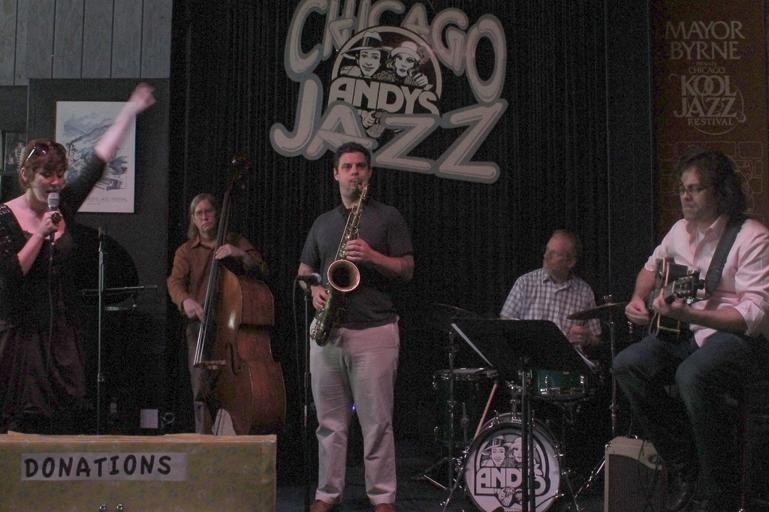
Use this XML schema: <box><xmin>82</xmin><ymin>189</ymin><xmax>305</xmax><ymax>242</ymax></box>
<box><xmin>411</xmin><ymin>322</ymin><xmax>619</xmax><ymax>512</ymax></box>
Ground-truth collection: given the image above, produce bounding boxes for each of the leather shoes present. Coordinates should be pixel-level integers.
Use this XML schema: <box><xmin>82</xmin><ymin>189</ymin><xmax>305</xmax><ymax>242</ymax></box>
<box><xmin>661</xmin><ymin>453</ymin><xmax>736</xmax><ymax>512</ymax></box>
<box><xmin>309</xmin><ymin>500</ymin><xmax>333</xmax><ymax>512</ymax></box>
<box><xmin>373</xmin><ymin>503</ymin><xmax>396</xmax><ymax>512</ymax></box>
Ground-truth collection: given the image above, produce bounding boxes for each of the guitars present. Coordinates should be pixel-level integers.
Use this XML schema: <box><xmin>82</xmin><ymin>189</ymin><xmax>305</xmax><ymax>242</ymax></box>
<box><xmin>639</xmin><ymin>257</ymin><xmax>707</xmax><ymax>344</ymax></box>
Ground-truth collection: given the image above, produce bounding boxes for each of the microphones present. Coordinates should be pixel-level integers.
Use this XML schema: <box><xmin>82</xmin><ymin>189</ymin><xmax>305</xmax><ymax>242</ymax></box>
<box><xmin>48</xmin><ymin>192</ymin><xmax>60</xmax><ymax>249</ymax></box>
<box><xmin>297</xmin><ymin>272</ymin><xmax>322</xmax><ymax>286</ymax></box>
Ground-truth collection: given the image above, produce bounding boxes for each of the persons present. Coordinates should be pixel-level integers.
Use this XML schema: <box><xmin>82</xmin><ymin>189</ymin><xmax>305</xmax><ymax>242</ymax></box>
<box><xmin>0</xmin><ymin>78</ymin><xmax>158</xmax><ymax>436</ymax></box>
<box><xmin>607</xmin><ymin>148</ymin><xmax>768</xmax><ymax>510</ymax></box>
<box><xmin>165</xmin><ymin>190</ymin><xmax>272</xmax><ymax>436</ymax></box>
<box><xmin>497</xmin><ymin>230</ymin><xmax>604</xmax><ymax>360</ymax></box>
<box><xmin>339</xmin><ymin>31</ymin><xmax>394</xmax><ymax>130</ymax></box>
<box><xmin>372</xmin><ymin>39</ymin><xmax>429</xmax><ymax>88</ymax></box>
<box><xmin>296</xmin><ymin>141</ymin><xmax>417</xmax><ymax>511</ymax></box>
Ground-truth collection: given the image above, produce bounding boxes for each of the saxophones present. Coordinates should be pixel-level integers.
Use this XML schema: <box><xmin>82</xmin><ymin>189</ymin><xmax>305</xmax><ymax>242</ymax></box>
<box><xmin>309</xmin><ymin>178</ymin><xmax>372</xmax><ymax>346</ymax></box>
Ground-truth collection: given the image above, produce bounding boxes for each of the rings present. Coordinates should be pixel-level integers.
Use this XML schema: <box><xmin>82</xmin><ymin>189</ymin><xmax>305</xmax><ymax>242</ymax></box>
<box><xmin>356</xmin><ymin>250</ymin><xmax>360</xmax><ymax>257</ymax></box>
<box><xmin>224</xmin><ymin>250</ymin><xmax>227</xmax><ymax>254</ymax></box>
<box><xmin>51</xmin><ymin>212</ymin><xmax>61</xmax><ymax>225</ymax></box>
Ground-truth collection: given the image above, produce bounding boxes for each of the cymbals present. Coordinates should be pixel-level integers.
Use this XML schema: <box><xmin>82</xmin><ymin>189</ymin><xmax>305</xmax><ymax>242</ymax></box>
<box><xmin>568</xmin><ymin>303</ymin><xmax>630</xmax><ymax>319</ymax></box>
<box><xmin>433</xmin><ymin>303</ymin><xmax>484</xmax><ymax>321</ymax></box>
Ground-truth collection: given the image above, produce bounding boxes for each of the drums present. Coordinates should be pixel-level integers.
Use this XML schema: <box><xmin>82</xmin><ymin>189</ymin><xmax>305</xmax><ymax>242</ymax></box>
<box><xmin>459</xmin><ymin>408</ymin><xmax>594</xmax><ymax>512</ymax></box>
<box><xmin>528</xmin><ymin>367</ymin><xmax>593</xmax><ymax>404</ymax></box>
<box><xmin>453</xmin><ymin>368</ymin><xmax>490</xmax><ymax>403</ymax></box>
<box><xmin>432</xmin><ymin>368</ymin><xmax>458</xmax><ymax>402</ymax></box>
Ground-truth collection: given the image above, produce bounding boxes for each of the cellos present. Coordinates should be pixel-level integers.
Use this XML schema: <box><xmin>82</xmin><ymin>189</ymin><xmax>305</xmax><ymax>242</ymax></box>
<box><xmin>193</xmin><ymin>152</ymin><xmax>287</xmax><ymax>435</ymax></box>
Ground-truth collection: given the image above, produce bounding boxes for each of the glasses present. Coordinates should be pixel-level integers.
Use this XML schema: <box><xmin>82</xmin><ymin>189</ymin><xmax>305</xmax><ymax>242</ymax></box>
<box><xmin>193</xmin><ymin>208</ymin><xmax>216</xmax><ymax>216</ymax></box>
<box><xmin>25</xmin><ymin>143</ymin><xmax>66</xmax><ymax>162</ymax></box>
<box><xmin>678</xmin><ymin>183</ymin><xmax>712</xmax><ymax>193</ymax></box>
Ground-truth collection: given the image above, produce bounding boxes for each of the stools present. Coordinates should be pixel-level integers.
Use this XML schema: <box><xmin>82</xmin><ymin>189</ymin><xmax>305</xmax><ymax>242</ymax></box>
<box><xmin>670</xmin><ymin>378</ymin><xmax>768</xmax><ymax>512</ymax></box>
